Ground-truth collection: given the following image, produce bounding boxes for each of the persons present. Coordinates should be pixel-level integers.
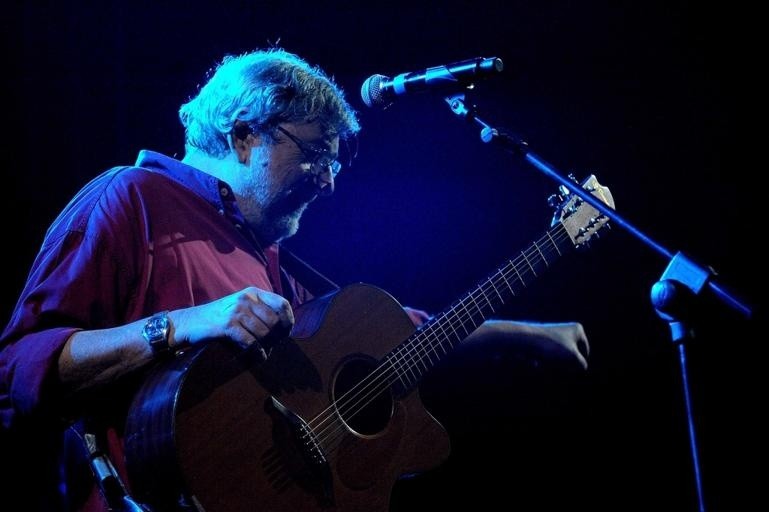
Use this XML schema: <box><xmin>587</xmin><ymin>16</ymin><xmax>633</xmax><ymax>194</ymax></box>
<box><xmin>1</xmin><ymin>44</ymin><xmax>595</xmax><ymax>511</ymax></box>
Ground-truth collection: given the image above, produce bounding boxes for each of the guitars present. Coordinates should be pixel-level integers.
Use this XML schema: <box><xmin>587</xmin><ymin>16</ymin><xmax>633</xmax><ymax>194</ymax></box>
<box><xmin>124</xmin><ymin>174</ymin><xmax>616</xmax><ymax>512</ymax></box>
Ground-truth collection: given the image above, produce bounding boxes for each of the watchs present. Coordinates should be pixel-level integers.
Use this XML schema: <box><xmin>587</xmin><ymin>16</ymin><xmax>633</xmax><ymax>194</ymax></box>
<box><xmin>142</xmin><ymin>306</ymin><xmax>173</xmax><ymax>363</ymax></box>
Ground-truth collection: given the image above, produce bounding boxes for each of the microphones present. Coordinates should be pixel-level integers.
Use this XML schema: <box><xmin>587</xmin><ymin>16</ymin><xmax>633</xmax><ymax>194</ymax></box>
<box><xmin>360</xmin><ymin>55</ymin><xmax>503</xmax><ymax>112</ymax></box>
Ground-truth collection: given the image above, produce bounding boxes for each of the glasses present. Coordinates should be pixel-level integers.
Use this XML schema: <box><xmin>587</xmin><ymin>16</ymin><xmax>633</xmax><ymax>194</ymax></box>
<box><xmin>277</xmin><ymin>124</ymin><xmax>341</xmax><ymax>179</ymax></box>
